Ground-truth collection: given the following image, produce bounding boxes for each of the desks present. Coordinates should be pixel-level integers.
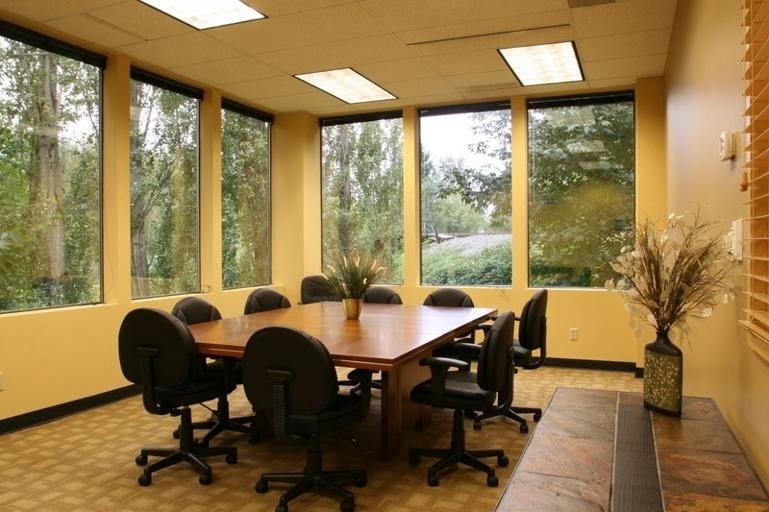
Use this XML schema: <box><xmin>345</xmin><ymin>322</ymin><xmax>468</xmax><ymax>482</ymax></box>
<box><xmin>495</xmin><ymin>385</ymin><xmax>768</xmax><ymax>510</ymax></box>
<box><xmin>186</xmin><ymin>299</ymin><xmax>497</xmax><ymax>463</ymax></box>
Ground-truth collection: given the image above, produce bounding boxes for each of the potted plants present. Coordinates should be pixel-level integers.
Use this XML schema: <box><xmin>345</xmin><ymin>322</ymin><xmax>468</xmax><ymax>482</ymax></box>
<box><xmin>600</xmin><ymin>200</ymin><xmax>748</xmax><ymax>419</ymax></box>
<box><xmin>328</xmin><ymin>253</ymin><xmax>388</xmax><ymax>318</ymax></box>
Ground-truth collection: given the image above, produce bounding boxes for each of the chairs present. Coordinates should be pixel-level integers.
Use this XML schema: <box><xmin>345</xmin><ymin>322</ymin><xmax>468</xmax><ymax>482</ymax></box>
<box><xmin>168</xmin><ymin>296</ymin><xmax>256</xmax><ymax>446</ymax></box>
<box><xmin>449</xmin><ymin>289</ymin><xmax>548</xmax><ymax>434</ymax></box>
<box><xmin>421</xmin><ymin>288</ymin><xmax>477</xmax><ymax>418</ymax></box>
<box><xmin>238</xmin><ymin>327</ymin><xmax>368</xmax><ymax>512</ymax></box>
<box><xmin>119</xmin><ymin>308</ymin><xmax>238</xmax><ymax>489</ymax></box>
<box><xmin>337</xmin><ymin>286</ymin><xmax>402</xmax><ymax>397</ymax></box>
<box><xmin>300</xmin><ymin>276</ymin><xmax>339</xmax><ymax>303</ymax></box>
<box><xmin>410</xmin><ymin>311</ymin><xmax>515</xmax><ymax>488</ymax></box>
<box><xmin>244</xmin><ymin>288</ymin><xmax>293</xmax><ymax>317</ymax></box>
